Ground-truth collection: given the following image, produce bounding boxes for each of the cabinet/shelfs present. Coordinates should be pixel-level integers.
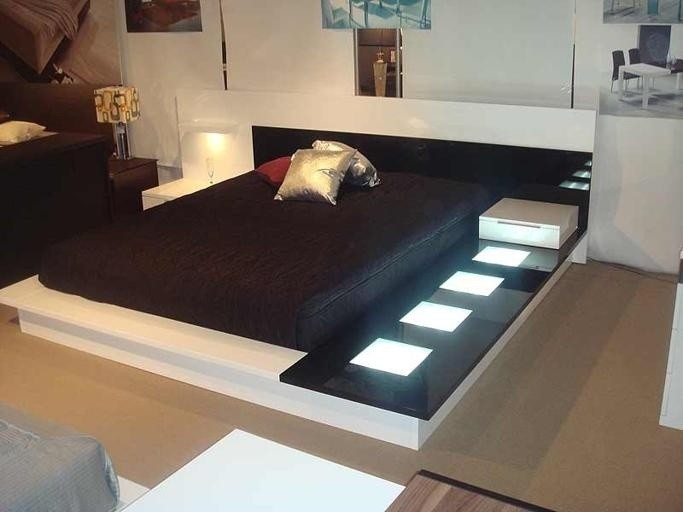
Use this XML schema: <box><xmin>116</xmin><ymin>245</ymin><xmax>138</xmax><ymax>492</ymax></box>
<box><xmin>659</xmin><ymin>250</ymin><xmax>683</xmax><ymax>430</ymax></box>
<box><xmin>638</xmin><ymin>25</ymin><xmax>671</xmax><ymax>68</ymax></box>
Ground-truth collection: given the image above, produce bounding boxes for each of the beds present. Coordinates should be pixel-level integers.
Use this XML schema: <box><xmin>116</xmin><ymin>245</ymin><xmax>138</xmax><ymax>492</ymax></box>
<box><xmin>1</xmin><ymin>88</ymin><xmax>597</xmax><ymax>451</ymax></box>
<box><xmin>1</xmin><ymin>405</ymin><xmax>121</xmax><ymax>511</ymax></box>
<box><xmin>0</xmin><ymin>0</ymin><xmax>87</xmax><ymax>74</ymax></box>
<box><xmin>0</xmin><ymin>84</ymin><xmax>121</xmax><ymax>166</ymax></box>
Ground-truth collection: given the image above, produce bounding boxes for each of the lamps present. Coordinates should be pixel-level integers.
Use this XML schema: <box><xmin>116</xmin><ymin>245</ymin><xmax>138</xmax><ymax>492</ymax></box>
<box><xmin>92</xmin><ymin>84</ymin><xmax>142</xmax><ymax>159</ymax></box>
<box><xmin>180</xmin><ymin>91</ymin><xmax>236</xmax><ymax>184</ymax></box>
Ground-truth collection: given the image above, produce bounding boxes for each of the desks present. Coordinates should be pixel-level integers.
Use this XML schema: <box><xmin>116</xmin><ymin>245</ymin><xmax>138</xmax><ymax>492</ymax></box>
<box><xmin>386</xmin><ymin>469</ymin><xmax>557</xmax><ymax>512</ymax></box>
<box><xmin>618</xmin><ymin>57</ymin><xmax>683</xmax><ymax>109</ymax></box>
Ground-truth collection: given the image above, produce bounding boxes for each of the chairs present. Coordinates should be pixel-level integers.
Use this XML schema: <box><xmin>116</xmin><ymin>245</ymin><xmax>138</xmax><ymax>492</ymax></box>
<box><xmin>322</xmin><ymin>0</ymin><xmax>351</xmax><ymax>28</ymax></box>
<box><xmin>610</xmin><ymin>48</ymin><xmax>638</xmax><ymax>94</ymax></box>
<box><xmin>629</xmin><ymin>48</ymin><xmax>654</xmax><ymax>89</ymax></box>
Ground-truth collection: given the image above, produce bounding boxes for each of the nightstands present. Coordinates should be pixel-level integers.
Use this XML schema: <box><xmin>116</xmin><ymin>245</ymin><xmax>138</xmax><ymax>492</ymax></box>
<box><xmin>108</xmin><ymin>158</ymin><xmax>159</xmax><ymax>222</ymax></box>
<box><xmin>142</xmin><ymin>178</ymin><xmax>229</xmax><ymax>210</ymax></box>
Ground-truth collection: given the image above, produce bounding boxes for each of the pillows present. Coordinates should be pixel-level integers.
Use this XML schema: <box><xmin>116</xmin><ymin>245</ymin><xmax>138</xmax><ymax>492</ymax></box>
<box><xmin>255</xmin><ymin>139</ymin><xmax>381</xmax><ymax>205</ymax></box>
<box><xmin>1</xmin><ymin>120</ymin><xmax>47</xmax><ymax>146</ymax></box>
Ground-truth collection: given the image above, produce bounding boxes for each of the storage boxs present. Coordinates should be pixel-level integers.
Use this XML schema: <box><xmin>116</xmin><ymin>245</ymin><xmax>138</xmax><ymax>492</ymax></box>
<box><xmin>478</xmin><ymin>197</ymin><xmax>579</xmax><ymax>249</ymax></box>
<box><xmin>477</xmin><ymin>235</ymin><xmax>579</xmax><ymax>272</ymax></box>
